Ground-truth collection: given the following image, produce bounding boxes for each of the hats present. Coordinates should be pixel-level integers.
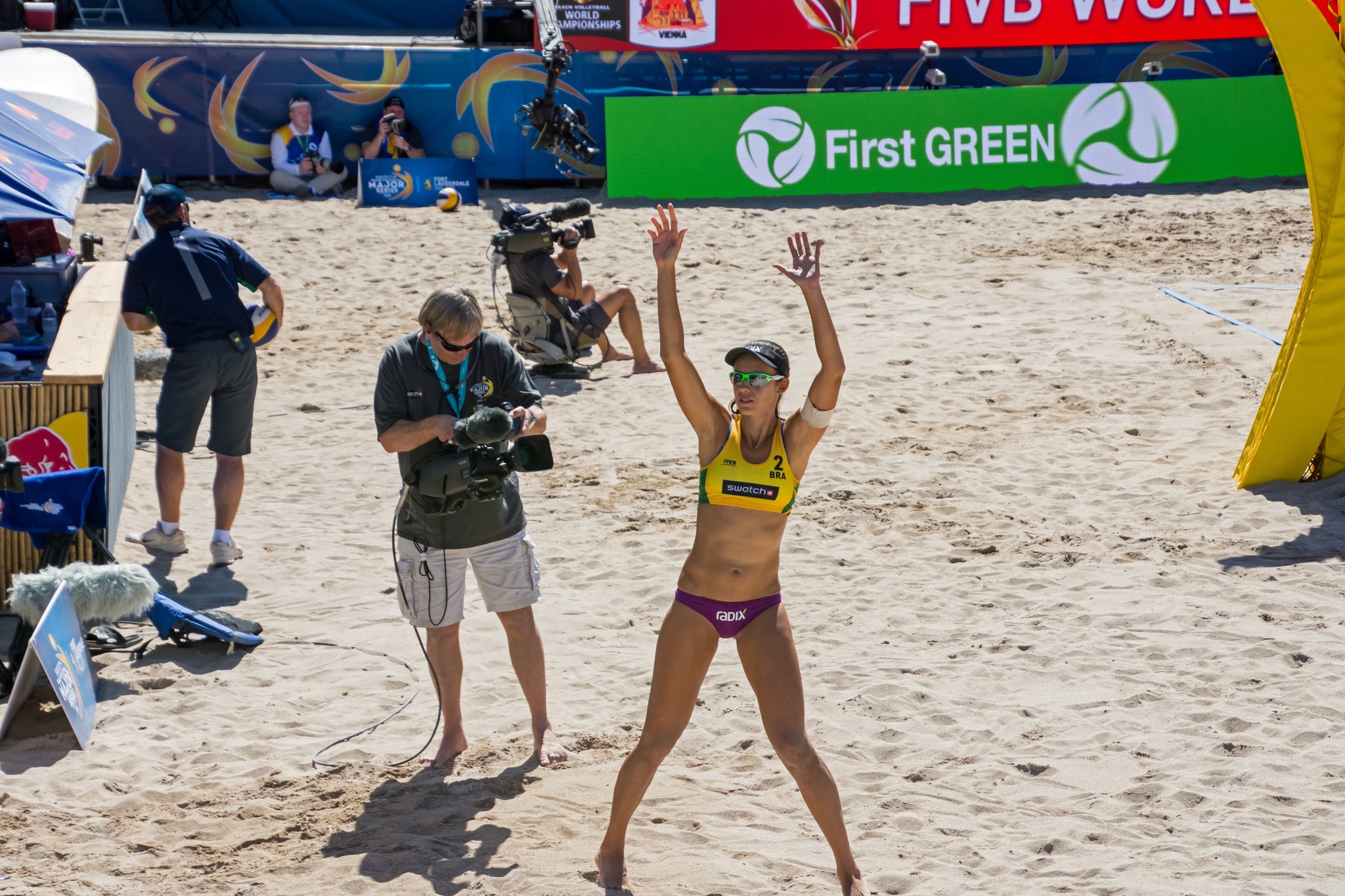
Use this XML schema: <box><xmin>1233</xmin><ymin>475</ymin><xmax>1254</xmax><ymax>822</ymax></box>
<box><xmin>289</xmin><ymin>96</ymin><xmax>310</xmax><ymax>109</ymax></box>
<box><xmin>142</xmin><ymin>183</ymin><xmax>195</xmax><ymax>219</ymax></box>
<box><xmin>725</xmin><ymin>344</ymin><xmax>790</xmax><ymax>378</ymax></box>
<box><xmin>384</xmin><ymin>96</ymin><xmax>404</xmax><ymax>108</ymax></box>
<box><xmin>498</xmin><ymin>203</ymin><xmax>532</xmax><ymax>231</ymax></box>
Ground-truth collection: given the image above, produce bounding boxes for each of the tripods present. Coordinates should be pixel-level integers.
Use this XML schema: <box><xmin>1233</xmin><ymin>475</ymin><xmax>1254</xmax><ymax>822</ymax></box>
<box><xmin>7</xmin><ymin>519</ymin><xmax>194</xmax><ymax>670</ymax></box>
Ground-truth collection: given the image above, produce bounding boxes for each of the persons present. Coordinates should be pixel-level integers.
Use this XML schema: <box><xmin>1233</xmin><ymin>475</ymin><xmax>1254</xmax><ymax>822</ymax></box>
<box><xmin>267</xmin><ymin>95</ymin><xmax>349</xmax><ymax>202</ymax></box>
<box><xmin>490</xmin><ymin>194</ymin><xmax>670</xmax><ymax>373</ymax></box>
<box><xmin>371</xmin><ymin>285</ymin><xmax>570</xmax><ymax>770</ymax></box>
<box><xmin>361</xmin><ymin>95</ymin><xmax>426</xmax><ymax>158</ymax></box>
<box><xmin>119</xmin><ymin>184</ymin><xmax>285</xmax><ymax>567</ymax></box>
<box><xmin>593</xmin><ymin>202</ymin><xmax>863</xmax><ymax>896</ymax></box>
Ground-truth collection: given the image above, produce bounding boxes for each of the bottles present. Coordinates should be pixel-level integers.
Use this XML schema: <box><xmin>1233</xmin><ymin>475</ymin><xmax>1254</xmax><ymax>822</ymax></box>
<box><xmin>40</xmin><ymin>302</ymin><xmax>58</xmax><ymax>353</ymax></box>
<box><xmin>11</xmin><ymin>280</ymin><xmax>29</xmax><ymax>329</ymax></box>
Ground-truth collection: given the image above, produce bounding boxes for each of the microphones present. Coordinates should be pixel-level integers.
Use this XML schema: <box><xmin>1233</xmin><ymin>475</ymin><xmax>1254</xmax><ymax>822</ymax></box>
<box><xmin>444</xmin><ymin>407</ymin><xmax>514</xmax><ymax>451</ymax></box>
<box><xmin>7</xmin><ymin>559</ymin><xmax>162</xmax><ymax>630</ymax></box>
<box><xmin>535</xmin><ymin>197</ymin><xmax>591</xmax><ymax>223</ymax></box>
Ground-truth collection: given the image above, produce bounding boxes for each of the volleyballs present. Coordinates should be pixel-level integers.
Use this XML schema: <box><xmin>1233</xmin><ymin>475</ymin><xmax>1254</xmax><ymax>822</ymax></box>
<box><xmin>436</xmin><ymin>187</ymin><xmax>462</xmax><ymax>212</ymax></box>
<box><xmin>244</xmin><ymin>302</ymin><xmax>278</xmax><ymax>351</ymax></box>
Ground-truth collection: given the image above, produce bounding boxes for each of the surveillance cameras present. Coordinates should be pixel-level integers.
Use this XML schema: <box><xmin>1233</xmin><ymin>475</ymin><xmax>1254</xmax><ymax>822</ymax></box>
<box><xmin>1142</xmin><ymin>61</ymin><xmax>1163</xmax><ymax>76</ymax></box>
<box><xmin>920</xmin><ymin>40</ymin><xmax>940</xmax><ymax>56</ymax></box>
<box><xmin>925</xmin><ymin>69</ymin><xmax>946</xmax><ymax>85</ymax></box>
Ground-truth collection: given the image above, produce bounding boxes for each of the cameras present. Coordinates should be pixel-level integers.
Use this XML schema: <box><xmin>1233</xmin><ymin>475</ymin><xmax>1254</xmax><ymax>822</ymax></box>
<box><xmin>305</xmin><ymin>150</ymin><xmax>344</xmax><ymax>174</ymax></box>
<box><xmin>383</xmin><ymin>113</ymin><xmax>414</xmax><ymax>135</ymax></box>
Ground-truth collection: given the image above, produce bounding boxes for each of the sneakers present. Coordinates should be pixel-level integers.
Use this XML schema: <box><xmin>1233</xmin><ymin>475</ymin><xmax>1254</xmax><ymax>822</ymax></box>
<box><xmin>125</xmin><ymin>520</ymin><xmax>189</xmax><ymax>554</ymax></box>
<box><xmin>209</xmin><ymin>537</ymin><xmax>244</xmax><ymax>565</ymax></box>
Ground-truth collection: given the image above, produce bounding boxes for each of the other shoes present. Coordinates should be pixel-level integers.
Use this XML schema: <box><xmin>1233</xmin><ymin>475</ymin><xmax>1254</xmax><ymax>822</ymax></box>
<box><xmin>298</xmin><ymin>184</ymin><xmax>313</xmax><ymax>199</ymax></box>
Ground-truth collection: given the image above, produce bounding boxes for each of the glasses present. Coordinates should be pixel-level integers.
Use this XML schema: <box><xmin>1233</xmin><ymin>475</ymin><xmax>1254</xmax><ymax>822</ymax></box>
<box><xmin>428</xmin><ymin>322</ymin><xmax>479</xmax><ymax>352</ymax></box>
<box><xmin>729</xmin><ymin>371</ymin><xmax>785</xmax><ymax>387</ymax></box>
<box><xmin>185</xmin><ymin>203</ymin><xmax>189</xmax><ymax>212</ymax></box>
